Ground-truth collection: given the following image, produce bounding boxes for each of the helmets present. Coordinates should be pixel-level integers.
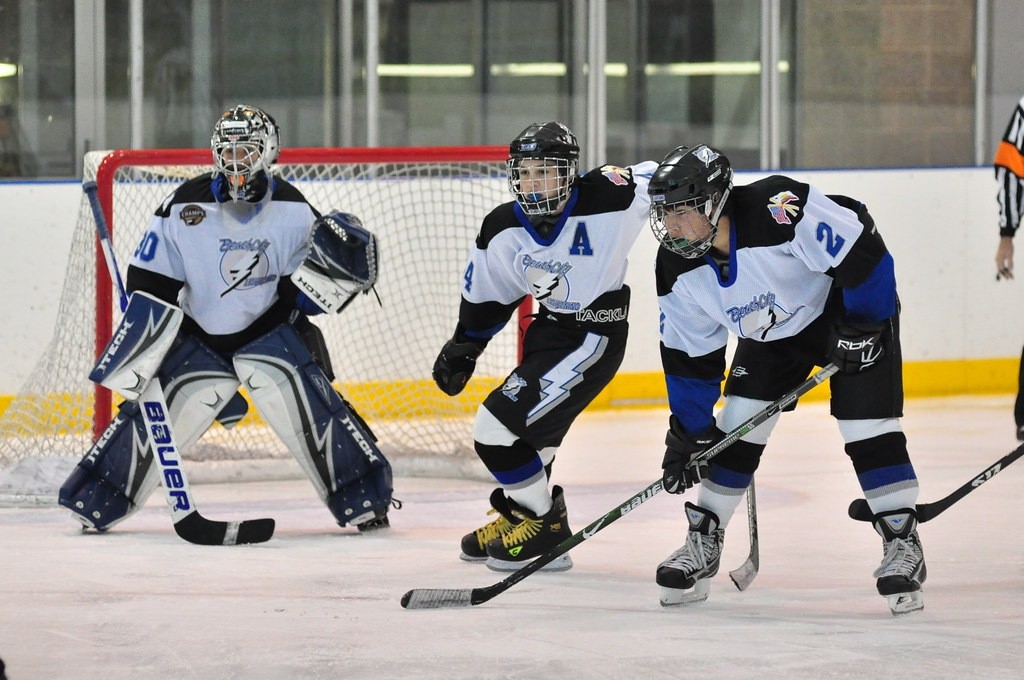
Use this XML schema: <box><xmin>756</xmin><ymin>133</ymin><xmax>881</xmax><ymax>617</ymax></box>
<box><xmin>210</xmin><ymin>104</ymin><xmax>280</xmax><ymax>205</ymax></box>
<box><xmin>647</xmin><ymin>143</ymin><xmax>734</xmax><ymax>258</ymax></box>
<box><xmin>504</xmin><ymin>120</ymin><xmax>580</xmax><ymax>216</ymax></box>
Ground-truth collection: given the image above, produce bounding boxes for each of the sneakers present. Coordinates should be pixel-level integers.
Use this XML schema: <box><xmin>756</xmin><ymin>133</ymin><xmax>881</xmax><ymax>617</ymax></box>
<box><xmin>872</xmin><ymin>507</ymin><xmax>927</xmax><ymax>618</ymax></box>
<box><xmin>460</xmin><ymin>488</ymin><xmax>527</xmax><ymax>560</ymax></box>
<box><xmin>486</xmin><ymin>485</ymin><xmax>573</xmax><ymax>572</ymax></box>
<box><xmin>656</xmin><ymin>501</ymin><xmax>724</xmax><ymax>607</ymax></box>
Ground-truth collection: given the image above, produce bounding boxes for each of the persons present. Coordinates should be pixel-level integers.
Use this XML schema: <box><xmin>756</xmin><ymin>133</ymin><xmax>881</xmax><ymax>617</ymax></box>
<box><xmin>56</xmin><ymin>105</ymin><xmax>403</xmax><ymax>535</ymax></box>
<box><xmin>433</xmin><ymin>122</ymin><xmax>662</xmax><ymax>573</ymax></box>
<box><xmin>994</xmin><ymin>94</ymin><xmax>1024</xmax><ymax>443</ymax></box>
<box><xmin>647</xmin><ymin>144</ymin><xmax>928</xmax><ymax>617</ymax></box>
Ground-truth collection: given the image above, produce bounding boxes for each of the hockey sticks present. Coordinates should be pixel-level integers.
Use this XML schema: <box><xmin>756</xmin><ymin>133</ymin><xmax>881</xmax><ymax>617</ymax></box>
<box><xmin>83</xmin><ymin>180</ymin><xmax>275</xmax><ymax>546</ymax></box>
<box><xmin>847</xmin><ymin>444</ymin><xmax>1024</xmax><ymax>525</ymax></box>
<box><xmin>400</xmin><ymin>364</ymin><xmax>837</xmax><ymax>609</ymax></box>
<box><xmin>727</xmin><ymin>474</ymin><xmax>761</xmax><ymax>590</ymax></box>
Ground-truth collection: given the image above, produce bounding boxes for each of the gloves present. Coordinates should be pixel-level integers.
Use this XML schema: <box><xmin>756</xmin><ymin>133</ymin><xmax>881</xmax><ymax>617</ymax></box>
<box><xmin>825</xmin><ymin>314</ymin><xmax>888</xmax><ymax>376</ymax></box>
<box><xmin>661</xmin><ymin>414</ymin><xmax>718</xmax><ymax>494</ymax></box>
<box><xmin>432</xmin><ymin>330</ymin><xmax>492</xmax><ymax>396</ymax></box>
